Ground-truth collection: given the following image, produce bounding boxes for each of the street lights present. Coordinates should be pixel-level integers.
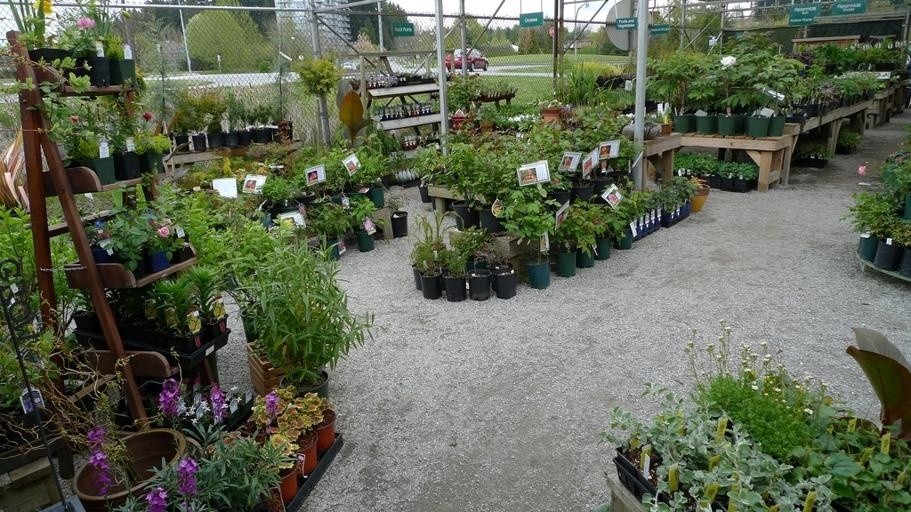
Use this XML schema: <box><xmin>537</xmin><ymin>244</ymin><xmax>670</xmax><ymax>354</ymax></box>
<box><xmin>574</xmin><ymin>3</ymin><xmax>590</xmax><ymax>63</ymax></box>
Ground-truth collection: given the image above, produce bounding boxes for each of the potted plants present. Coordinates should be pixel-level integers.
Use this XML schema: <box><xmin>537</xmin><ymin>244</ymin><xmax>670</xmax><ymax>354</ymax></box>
<box><xmin>606</xmin><ymin>317</ymin><xmax>911</xmax><ymax>511</ymax></box>
<box><xmin>557</xmin><ymin>43</ymin><xmax>911</xmax><ymax>193</ymax></box>
<box><xmin>0</xmin><ymin>0</ymin><xmax>386</xmax><ymax>512</ymax></box>
<box><xmin>839</xmin><ymin>156</ymin><xmax>911</xmax><ymax>286</ymax></box>
<box><xmin>385</xmin><ymin>74</ymin><xmax>705</xmax><ymax>304</ymax></box>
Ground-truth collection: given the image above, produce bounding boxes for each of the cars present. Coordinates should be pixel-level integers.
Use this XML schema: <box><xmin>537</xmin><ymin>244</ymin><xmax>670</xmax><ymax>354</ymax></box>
<box><xmin>446</xmin><ymin>48</ymin><xmax>489</xmax><ymax>72</ymax></box>
